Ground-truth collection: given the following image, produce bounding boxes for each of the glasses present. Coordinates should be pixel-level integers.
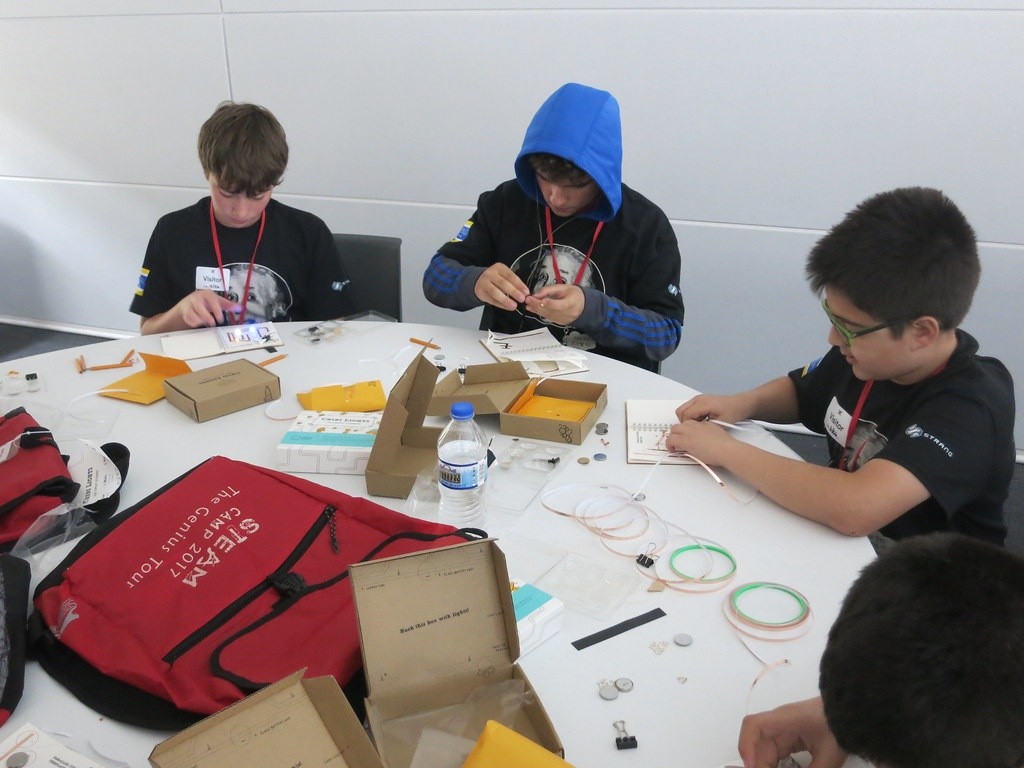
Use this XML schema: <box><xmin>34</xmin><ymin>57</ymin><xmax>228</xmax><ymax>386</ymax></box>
<box><xmin>821</xmin><ymin>298</ymin><xmax>942</xmax><ymax>347</ymax></box>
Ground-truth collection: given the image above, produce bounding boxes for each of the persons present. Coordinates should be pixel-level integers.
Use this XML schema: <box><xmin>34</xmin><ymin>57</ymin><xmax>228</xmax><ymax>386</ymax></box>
<box><xmin>422</xmin><ymin>83</ymin><xmax>686</xmax><ymax>373</ymax></box>
<box><xmin>129</xmin><ymin>100</ymin><xmax>358</xmax><ymax>336</ymax></box>
<box><xmin>666</xmin><ymin>188</ymin><xmax>1016</xmax><ymax>556</ymax></box>
<box><xmin>739</xmin><ymin>529</ymin><xmax>1024</xmax><ymax>768</ymax></box>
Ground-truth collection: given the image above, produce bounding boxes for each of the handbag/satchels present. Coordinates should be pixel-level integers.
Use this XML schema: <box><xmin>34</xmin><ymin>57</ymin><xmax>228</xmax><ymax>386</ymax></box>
<box><xmin>0</xmin><ymin>555</ymin><xmax>32</xmax><ymax>728</ymax></box>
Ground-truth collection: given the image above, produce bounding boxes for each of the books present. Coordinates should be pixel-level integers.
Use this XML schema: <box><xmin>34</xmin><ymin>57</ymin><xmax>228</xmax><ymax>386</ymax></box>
<box><xmin>159</xmin><ymin>321</ymin><xmax>285</xmax><ymax>361</ymax></box>
<box><xmin>479</xmin><ymin>327</ymin><xmax>589</xmax><ymax>383</ymax></box>
<box><xmin>625</xmin><ymin>398</ymin><xmax>721</xmax><ymax>464</ymax></box>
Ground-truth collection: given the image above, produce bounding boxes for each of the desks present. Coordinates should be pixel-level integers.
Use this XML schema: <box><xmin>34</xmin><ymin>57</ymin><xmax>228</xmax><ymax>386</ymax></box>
<box><xmin>0</xmin><ymin>320</ymin><xmax>877</xmax><ymax>768</ymax></box>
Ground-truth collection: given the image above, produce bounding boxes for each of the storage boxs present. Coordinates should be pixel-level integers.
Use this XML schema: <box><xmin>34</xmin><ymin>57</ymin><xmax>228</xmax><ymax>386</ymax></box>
<box><xmin>365</xmin><ymin>340</ymin><xmax>467</xmax><ymax>503</ymax></box>
<box><xmin>276</xmin><ymin>410</ymin><xmax>384</xmax><ymax>475</ymax></box>
<box><xmin>147</xmin><ymin>668</ymin><xmax>383</xmax><ymax>768</ymax></box>
<box><xmin>426</xmin><ymin>361</ymin><xmax>607</xmax><ymax>445</ymax></box>
<box><xmin>347</xmin><ymin>537</ymin><xmax>564</xmax><ymax>768</ymax></box>
<box><xmin>163</xmin><ymin>358</ymin><xmax>280</xmax><ymax>423</ymax></box>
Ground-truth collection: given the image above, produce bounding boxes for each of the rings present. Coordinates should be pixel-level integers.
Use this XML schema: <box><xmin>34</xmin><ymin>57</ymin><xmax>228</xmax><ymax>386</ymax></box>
<box><xmin>537</xmin><ymin>300</ymin><xmax>547</xmax><ymax>310</ymax></box>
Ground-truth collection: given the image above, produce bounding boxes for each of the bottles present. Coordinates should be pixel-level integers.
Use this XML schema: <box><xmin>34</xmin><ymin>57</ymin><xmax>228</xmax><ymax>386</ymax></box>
<box><xmin>5</xmin><ymin>370</ymin><xmax>39</xmax><ymax>393</ymax></box>
<box><xmin>437</xmin><ymin>401</ymin><xmax>489</xmax><ymax>528</ymax></box>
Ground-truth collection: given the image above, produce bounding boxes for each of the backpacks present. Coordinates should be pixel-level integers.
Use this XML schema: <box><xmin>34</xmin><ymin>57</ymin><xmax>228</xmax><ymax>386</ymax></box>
<box><xmin>25</xmin><ymin>454</ymin><xmax>490</xmax><ymax>730</ymax></box>
<box><xmin>0</xmin><ymin>406</ymin><xmax>82</xmax><ymax>554</ymax></box>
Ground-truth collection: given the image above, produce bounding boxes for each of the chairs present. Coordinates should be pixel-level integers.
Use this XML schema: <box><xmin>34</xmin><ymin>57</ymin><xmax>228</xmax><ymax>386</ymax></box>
<box><xmin>331</xmin><ymin>234</ymin><xmax>401</xmax><ymax>321</ymax></box>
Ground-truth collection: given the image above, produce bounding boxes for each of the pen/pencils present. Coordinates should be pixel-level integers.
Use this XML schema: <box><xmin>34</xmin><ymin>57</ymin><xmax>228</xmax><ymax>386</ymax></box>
<box><xmin>258</xmin><ymin>353</ymin><xmax>289</xmax><ymax>367</ymax></box>
<box><xmin>74</xmin><ymin>348</ymin><xmax>136</xmax><ymax>373</ymax></box>
<box><xmin>410</xmin><ymin>338</ymin><xmax>441</xmax><ymax>349</ymax></box>
